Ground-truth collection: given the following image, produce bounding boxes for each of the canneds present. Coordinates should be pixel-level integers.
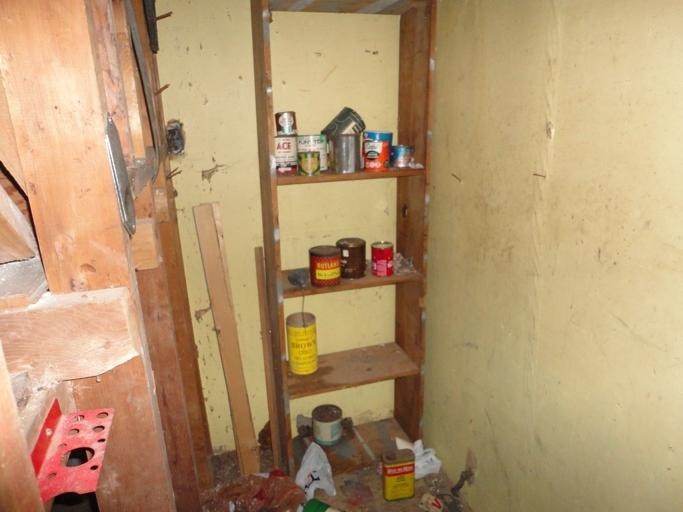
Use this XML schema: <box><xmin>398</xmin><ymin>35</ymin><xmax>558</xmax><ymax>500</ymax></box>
<box><xmin>312</xmin><ymin>404</ymin><xmax>344</xmax><ymax>446</ymax></box>
<box><xmin>286</xmin><ymin>312</ymin><xmax>317</xmax><ymax>375</ymax></box>
<box><xmin>371</xmin><ymin>242</ymin><xmax>395</xmax><ymax>277</ymax></box>
<box><xmin>273</xmin><ymin>107</ymin><xmax>414</xmax><ymax>178</ymax></box>
<box><xmin>310</xmin><ymin>246</ymin><xmax>342</xmax><ymax>287</ymax></box>
<box><xmin>336</xmin><ymin>237</ymin><xmax>365</xmax><ymax>278</ymax></box>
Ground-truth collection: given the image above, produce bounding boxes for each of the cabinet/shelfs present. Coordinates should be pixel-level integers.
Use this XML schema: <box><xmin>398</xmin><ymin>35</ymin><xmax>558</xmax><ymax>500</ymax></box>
<box><xmin>252</xmin><ymin>0</ymin><xmax>437</xmax><ymax>482</ymax></box>
